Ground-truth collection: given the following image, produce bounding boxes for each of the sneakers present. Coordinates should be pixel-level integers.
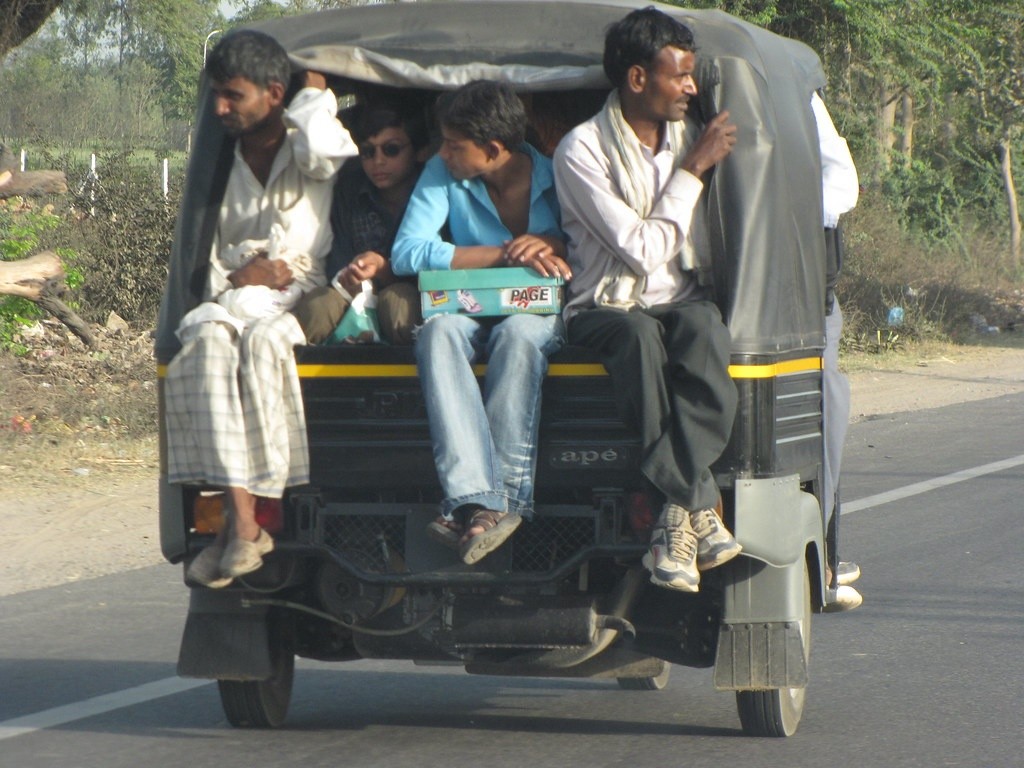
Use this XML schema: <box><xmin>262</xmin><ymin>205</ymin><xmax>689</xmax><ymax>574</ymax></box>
<box><xmin>689</xmin><ymin>502</ymin><xmax>743</xmax><ymax>572</ymax></box>
<box><xmin>642</xmin><ymin>519</ymin><xmax>700</xmax><ymax>592</ymax></box>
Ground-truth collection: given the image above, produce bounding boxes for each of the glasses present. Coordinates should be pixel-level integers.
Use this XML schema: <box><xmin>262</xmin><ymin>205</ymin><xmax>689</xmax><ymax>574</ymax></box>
<box><xmin>358</xmin><ymin>140</ymin><xmax>417</xmax><ymax>161</ymax></box>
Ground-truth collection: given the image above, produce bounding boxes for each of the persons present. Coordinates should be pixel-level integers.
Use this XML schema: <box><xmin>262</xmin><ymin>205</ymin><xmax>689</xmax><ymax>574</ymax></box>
<box><xmin>296</xmin><ymin>97</ymin><xmax>424</xmax><ymax>345</ymax></box>
<box><xmin>391</xmin><ymin>79</ymin><xmax>572</xmax><ymax>564</ymax></box>
<box><xmin>553</xmin><ymin>4</ymin><xmax>744</xmax><ymax>593</ymax></box>
<box><xmin>812</xmin><ymin>90</ymin><xmax>862</xmax><ymax>612</ymax></box>
<box><xmin>165</xmin><ymin>29</ymin><xmax>358</xmax><ymax>588</ymax></box>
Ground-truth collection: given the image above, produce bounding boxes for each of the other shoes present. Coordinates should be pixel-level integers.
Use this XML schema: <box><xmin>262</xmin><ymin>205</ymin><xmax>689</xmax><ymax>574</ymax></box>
<box><xmin>219</xmin><ymin>526</ymin><xmax>278</xmax><ymax>577</ymax></box>
<box><xmin>185</xmin><ymin>530</ymin><xmax>238</xmax><ymax>589</ymax></box>
<box><xmin>835</xmin><ymin>559</ymin><xmax>862</xmax><ymax>585</ymax></box>
<box><xmin>823</xmin><ymin>587</ymin><xmax>863</xmax><ymax>614</ymax></box>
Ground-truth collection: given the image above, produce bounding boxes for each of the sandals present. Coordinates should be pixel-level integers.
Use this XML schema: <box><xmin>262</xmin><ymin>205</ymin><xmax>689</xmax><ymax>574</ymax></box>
<box><xmin>427</xmin><ymin>514</ymin><xmax>463</xmax><ymax>550</ymax></box>
<box><xmin>460</xmin><ymin>507</ymin><xmax>522</xmax><ymax>566</ymax></box>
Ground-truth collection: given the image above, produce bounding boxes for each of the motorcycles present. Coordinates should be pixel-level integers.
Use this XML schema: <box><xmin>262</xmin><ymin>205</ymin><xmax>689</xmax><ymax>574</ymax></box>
<box><xmin>148</xmin><ymin>0</ymin><xmax>863</xmax><ymax>735</ymax></box>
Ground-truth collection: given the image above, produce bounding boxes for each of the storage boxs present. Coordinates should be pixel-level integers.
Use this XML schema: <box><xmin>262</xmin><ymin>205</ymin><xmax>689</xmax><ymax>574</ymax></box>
<box><xmin>418</xmin><ymin>266</ymin><xmax>568</xmax><ymax>318</ymax></box>
